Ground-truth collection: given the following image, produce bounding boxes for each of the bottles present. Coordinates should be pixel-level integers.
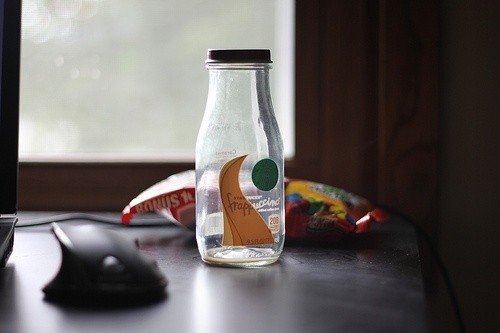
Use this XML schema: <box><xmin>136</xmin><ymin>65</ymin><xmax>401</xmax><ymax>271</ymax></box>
<box><xmin>194</xmin><ymin>49</ymin><xmax>285</xmax><ymax>268</ymax></box>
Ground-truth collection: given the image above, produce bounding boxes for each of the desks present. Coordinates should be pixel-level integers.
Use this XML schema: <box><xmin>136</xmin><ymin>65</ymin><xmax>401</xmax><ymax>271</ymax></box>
<box><xmin>0</xmin><ymin>209</ymin><xmax>428</xmax><ymax>333</ymax></box>
